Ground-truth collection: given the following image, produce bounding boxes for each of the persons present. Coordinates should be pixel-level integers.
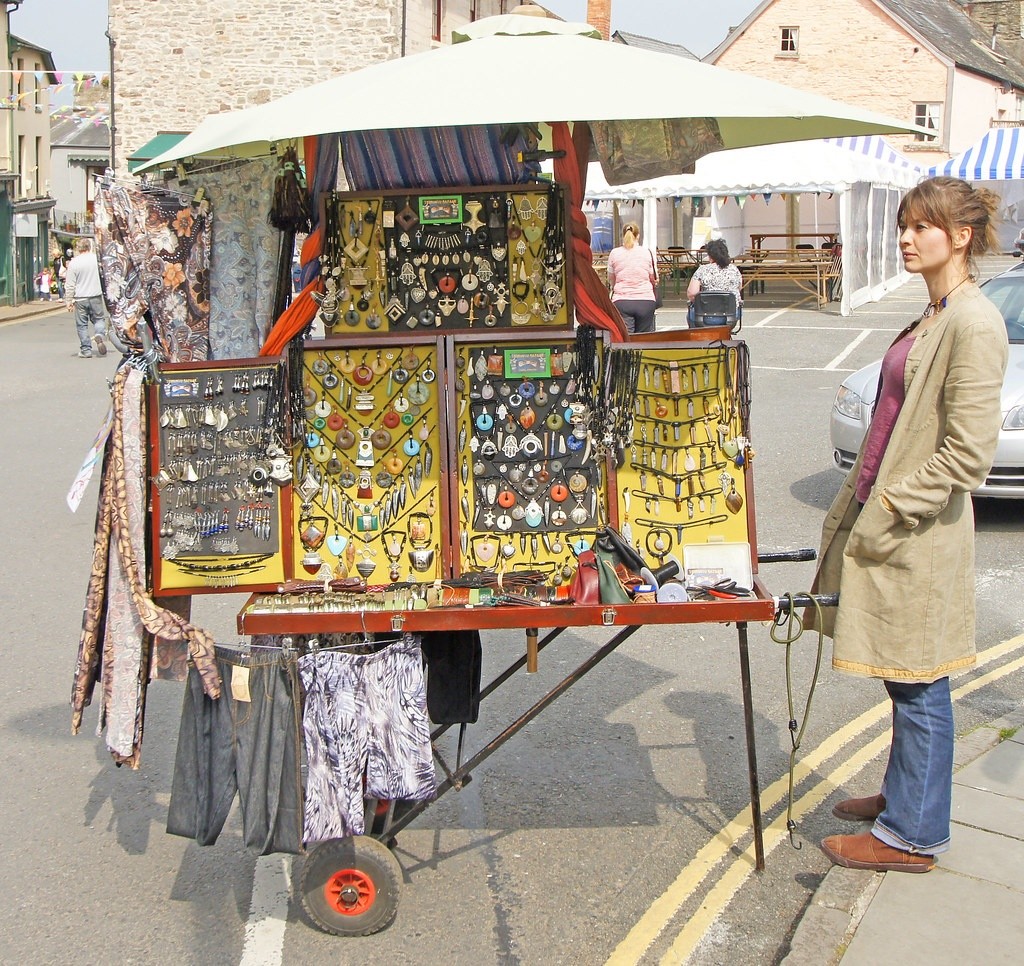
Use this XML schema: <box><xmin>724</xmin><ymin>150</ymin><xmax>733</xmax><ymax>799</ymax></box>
<box><xmin>801</xmin><ymin>177</ymin><xmax>1008</xmax><ymax>874</ymax></box>
<box><xmin>52</xmin><ymin>249</ymin><xmax>66</xmax><ymax>303</ymax></box>
<box><xmin>685</xmin><ymin>238</ymin><xmax>744</xmax><ymax>326</ymax></box>
<box><xmin>607</xmin><ymin>222</ymin><xmax>659</xmax><ymax>334</ymax></box>
<box><xmin>64</xmin><ymin>238</ymin><xmax>108</xmax><ymax>359</ymax></box>
<box><xmin>38</xmin><ymin>268</ymin><xmax>53</xmax><ymax>301</ymax></box>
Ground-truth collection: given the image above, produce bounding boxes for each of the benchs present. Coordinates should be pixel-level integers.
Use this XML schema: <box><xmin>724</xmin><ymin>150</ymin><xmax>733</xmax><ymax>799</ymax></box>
<box><xmin>742</xmin><ymin>269</ymin><xmax>818</xmax><ymax>273</ymax></box>
<box><xmin>742</xmin><ymin>277</ymin><xmax>829</xmax><ymax>280</ymax></box>
<box><xmin>757</xmin><ymin>267</ymin><xmax>816</xmax><ymax>270</ymax></box>
<box><xmin>741</xmin><ymin>274</ymin><xmax>841</xmax><ymax>277</ymax></box>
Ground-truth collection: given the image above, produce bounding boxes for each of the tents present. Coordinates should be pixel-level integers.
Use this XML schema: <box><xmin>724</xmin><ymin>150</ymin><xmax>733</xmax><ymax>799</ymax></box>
<box><xmin>586</xmin><ymin>134</ymin><xmax>927</xmax><ymax>318</ymax></box>
<box><xmin>923</xmin><ymin>126</ymin><xmax>1024</xmax><ymax>256</ymax></box>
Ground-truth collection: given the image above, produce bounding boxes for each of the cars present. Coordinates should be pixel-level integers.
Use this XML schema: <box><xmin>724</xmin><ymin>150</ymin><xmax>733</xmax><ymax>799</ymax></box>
<box><xmin>831</xmin><ymin>261</ymin><xmax>1023</xmax><ymax>501</ymax></box>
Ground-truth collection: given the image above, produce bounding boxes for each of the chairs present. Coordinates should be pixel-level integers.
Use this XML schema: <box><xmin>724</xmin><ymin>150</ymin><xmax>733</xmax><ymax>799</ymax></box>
<box><xmin>796</xmin><ymin>244</ymin><xmax>819</xmax><ymax>268</ymax></box>
<box><xmin>694</xmin><ymin>292</ymin><xmax>737</xmax><ymax>328</ymax></box>
<box><xmin>667</xmin><ymin>246</ymin><xmax>690</xmax><ymax>282</ymax></box>
<box><xmin>821</xmin><ymin>242</ymin><xmax>842</xmax><ymax>262</ymax></box>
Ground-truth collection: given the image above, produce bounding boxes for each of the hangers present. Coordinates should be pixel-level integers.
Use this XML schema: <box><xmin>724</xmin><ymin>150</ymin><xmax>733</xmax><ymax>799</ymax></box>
<box><xmin>90</xmin><ymin>165</ymin><xmax>210</xmax><ymax>208</ymax></box>
<box><xmin>306</xmin><ymin>608</ymin><xmax>414</xmax><ymax>660</ymax></box>
<box><xmin>174</xmin><ymin>143</ymin><xmax>279</xmax><ymax>187</ymax></box>
<box><xmin>186</xmin><ymin>611</ymin><xmax>300</xmax><ymax>663</ymax></box>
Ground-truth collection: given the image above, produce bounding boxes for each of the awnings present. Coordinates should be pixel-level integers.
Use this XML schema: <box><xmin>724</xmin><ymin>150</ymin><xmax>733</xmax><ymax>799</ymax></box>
<box><xmin>125</xmin><ymin>131</ymin><xmax>190</xmax><ymax>173</ymax></box>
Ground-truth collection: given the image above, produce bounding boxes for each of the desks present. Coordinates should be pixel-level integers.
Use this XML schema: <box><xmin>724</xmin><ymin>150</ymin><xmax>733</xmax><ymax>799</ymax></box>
<box><xmin>591</xmin><ymin>248</ymin><xmax>840</xmax><ymax>311</ymax></box>
<box><xmin>749</xmin><ymin>232</ymin><xmax>839</xmax><ymax>263</ymax></box>
<box><xmin>233</xmin><ymin>574</ymin><xmax>778</xmax><ymax>874</ymax></box>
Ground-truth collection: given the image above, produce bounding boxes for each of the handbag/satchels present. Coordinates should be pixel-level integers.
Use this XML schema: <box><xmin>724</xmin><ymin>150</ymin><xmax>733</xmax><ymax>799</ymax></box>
<box><xmin>59</xmin><ymin>257</ymin><xmax>66</xmax><ymax>279</ymax></box>
<box><xmin>648</xmin><ymin>249</ymin><xmax>663</xmax><ymax>309</ymax></box>
<box><xmin>571</xmin><ymin>525</ymin><xmax>654</xmax><ymax>605</ymax></box>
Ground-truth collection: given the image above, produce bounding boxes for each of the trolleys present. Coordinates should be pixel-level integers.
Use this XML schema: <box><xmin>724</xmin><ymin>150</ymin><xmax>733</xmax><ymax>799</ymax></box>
<box><xmin>235</xmin><ymin>544</ymin><xmax>843</xmax><ymax>937</ymax></box>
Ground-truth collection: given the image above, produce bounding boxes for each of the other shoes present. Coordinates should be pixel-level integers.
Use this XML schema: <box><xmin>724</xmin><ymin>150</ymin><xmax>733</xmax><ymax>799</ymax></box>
<box><xmin>95</xmin><ymin>333</ymin><xmax>107</xmax><ymax>355</ymax></box>
<box><xmin>832</xmin><ymin>794</ymin><xmax>886</xmax><ymax>820</ymax></box>
<box><xmin>49</xmin><ymin>298</ymin><xmax>52</xmax><ymax>302</ymax></box>
<box><xmin>821</xmin><ymin>832</ymin><xmax>934</xmax><ymax>872</ymax></box>
<box><xmin>78</xmin><ymin>349</ymin><xmax>92</xmax><ymax>358</ymax></box>
<box><xmin>40</xmin><ymin>298</ymin><xmax>44</xmax><ymax>302</ymax></box>
<box><xmin>58</xmin><ymin>298</ymin><xmax>63</xmax><ymax>303</ymax></box>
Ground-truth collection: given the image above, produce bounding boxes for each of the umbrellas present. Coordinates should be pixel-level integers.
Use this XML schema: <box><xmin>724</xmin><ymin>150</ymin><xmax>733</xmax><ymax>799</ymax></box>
<box><xmin>126</xmin><ymin>2</ymin><xmax>937</xmax><ymax>203</ymax></box>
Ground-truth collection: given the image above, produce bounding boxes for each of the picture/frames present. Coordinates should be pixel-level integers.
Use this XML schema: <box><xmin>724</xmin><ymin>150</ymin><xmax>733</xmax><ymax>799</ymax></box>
<box><xmin>418</xmin><ymin>195</ymin><xmax>463</xmax><ymax>224</ymax></box>
<box><xmin>504</xmin><ymin>348</ymin><xmax>551</xmax><ymax>378</ymax></box>
